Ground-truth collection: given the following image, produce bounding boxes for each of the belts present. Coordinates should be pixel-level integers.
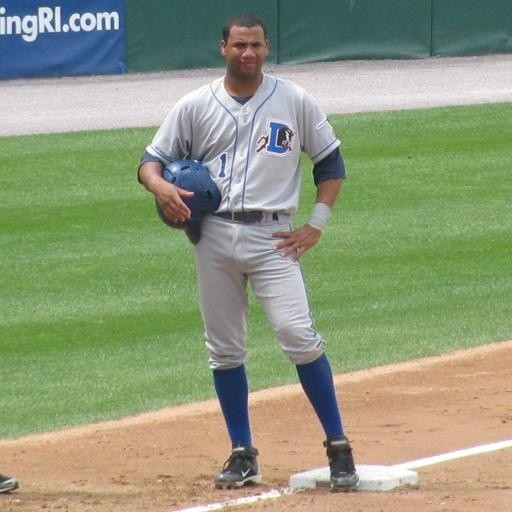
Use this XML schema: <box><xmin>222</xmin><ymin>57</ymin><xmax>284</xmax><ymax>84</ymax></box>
<box><xmin>211</xmin><ymin>210</ymin><xmax>278</xmax><ymax>223</ymax></box>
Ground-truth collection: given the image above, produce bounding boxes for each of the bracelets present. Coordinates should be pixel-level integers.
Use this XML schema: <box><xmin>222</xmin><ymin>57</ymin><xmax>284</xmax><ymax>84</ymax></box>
<box><xmin>307</xmin><ymin>203</ymin><xmax>332</xmax><ymax>234</ymax></box>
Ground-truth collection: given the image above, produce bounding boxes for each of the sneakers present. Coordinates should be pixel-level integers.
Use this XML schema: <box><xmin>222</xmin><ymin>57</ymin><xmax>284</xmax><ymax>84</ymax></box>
<box><xmin>213</xmin><ymin>447</ymin><xmax>262</xmax><ymax>489</ymax></box>
<box><xmin>323</xmin><ymin>437</ymin><xmax>361</xmax><ymax>492</ymax></box>
<box><xmin>0</xmin><ymin>475</ymin><xmax>19</xmax><ymax>494</ymax></box>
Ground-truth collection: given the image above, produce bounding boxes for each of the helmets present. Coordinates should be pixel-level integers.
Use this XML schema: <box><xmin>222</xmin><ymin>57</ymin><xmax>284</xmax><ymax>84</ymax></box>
<box><xmin>155</xmin><ymin>160</ymin><xmax>221</xmax><ymax>244</ymax></box>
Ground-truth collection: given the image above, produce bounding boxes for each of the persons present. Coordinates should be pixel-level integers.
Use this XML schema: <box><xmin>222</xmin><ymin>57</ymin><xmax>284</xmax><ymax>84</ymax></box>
<box><xmin>1</xmin><ymin>473</ymin><xmax>21</xmax><ymax>496</ymax></box>
<box><xmin>134</xmin><ymin>11</ymin><xmax>361</xmax><ymax>491</ymax></box>
